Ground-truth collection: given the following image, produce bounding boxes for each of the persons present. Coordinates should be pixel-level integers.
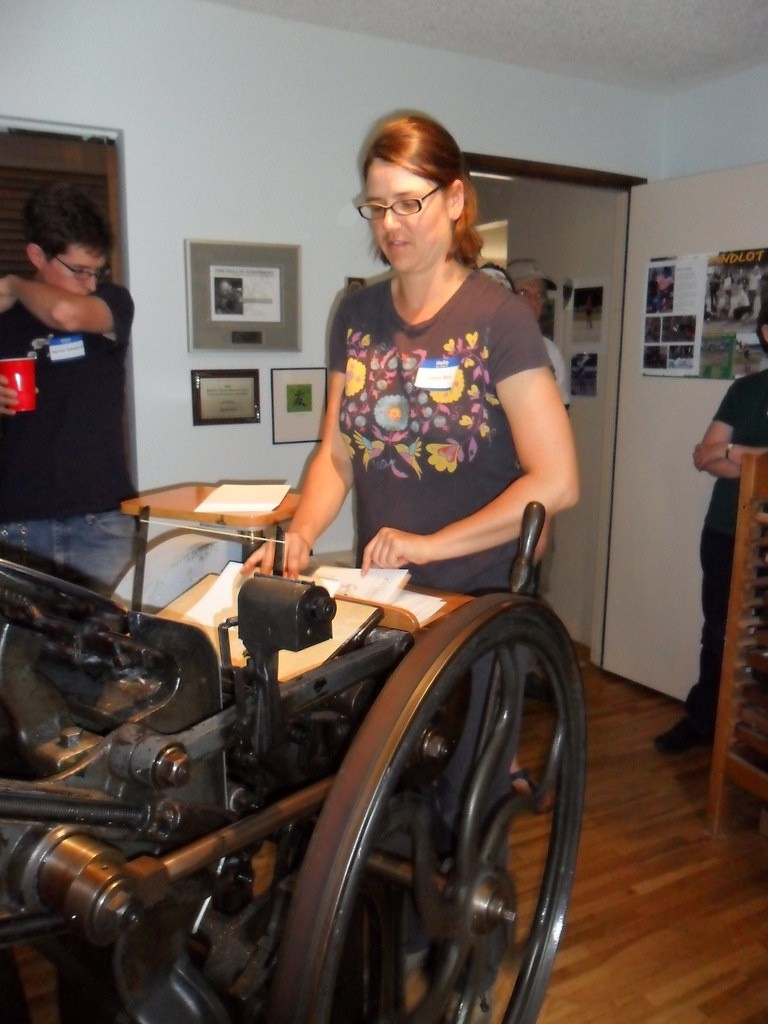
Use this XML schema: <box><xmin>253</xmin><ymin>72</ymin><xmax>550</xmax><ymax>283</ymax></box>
<box><xmin>241</xmin><ymin>108</ymin><xmax>578</xmax><ymax>794</ymax></box>
<box><xmin>709</xmin><ymin>264</ymin><xmax>762</xmax><ymax>321</ymax></box>
<box><xmin>215</xmin><ymin>281</ymin><xmax>243</xmax><ymax>314</ymax></box>
<box><xmin>0</xmin><ymin>183</ymin><xmax>135</xmax><ymax>524</ymax></box>
<box><xmin>647</xmin><ymin>264</ymin><xmax>674</xmax><ymax>313</ymax></box>
<box><xmin>650</xmin><ymin>291</ymin><xmax>767</xmax><ymax>756</ymax></box>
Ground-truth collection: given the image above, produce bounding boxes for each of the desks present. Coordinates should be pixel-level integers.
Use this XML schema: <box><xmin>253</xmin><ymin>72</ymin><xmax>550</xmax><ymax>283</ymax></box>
<box><xmin>120</xmin><ymin>485</ymin><xmax>302</xmax><ymax>615</ymax></box>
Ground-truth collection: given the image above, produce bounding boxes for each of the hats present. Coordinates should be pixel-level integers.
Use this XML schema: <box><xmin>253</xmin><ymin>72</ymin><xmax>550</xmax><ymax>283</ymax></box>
<box><xmin>505</xmin><ymin>258</ymin><xmax>559</xmax><ymax>290</ymax></box>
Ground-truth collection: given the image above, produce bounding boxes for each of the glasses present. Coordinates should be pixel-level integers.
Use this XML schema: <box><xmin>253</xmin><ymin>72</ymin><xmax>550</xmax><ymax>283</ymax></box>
<box><xmin>45</xmin><ymin>249</ymin><xmax>111</xmax><ymax>281</ymax></box>
<box><xmin>357</xmin><ymin>183</ymin><xmax>443</xmax><ymax>220</ymax></box>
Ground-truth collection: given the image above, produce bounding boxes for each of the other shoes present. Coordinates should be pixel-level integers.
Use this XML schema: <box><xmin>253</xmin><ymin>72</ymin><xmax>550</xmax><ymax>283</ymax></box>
<box><xmin>654</xmin><ymin>718</ymin><xmax>712</xmax><ymax>753</ymax></box>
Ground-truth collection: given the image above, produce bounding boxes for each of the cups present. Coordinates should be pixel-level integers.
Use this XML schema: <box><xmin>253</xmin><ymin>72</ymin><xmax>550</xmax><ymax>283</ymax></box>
<box><xmin>0</xmin><ymin>358</ymin><xmax>36</xmax><ymax>412</ymax></box>
